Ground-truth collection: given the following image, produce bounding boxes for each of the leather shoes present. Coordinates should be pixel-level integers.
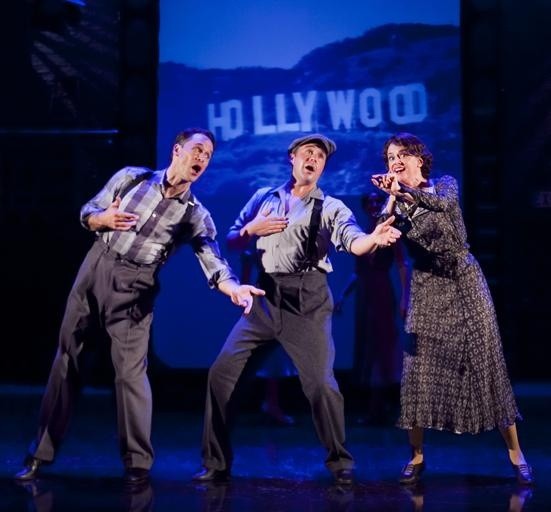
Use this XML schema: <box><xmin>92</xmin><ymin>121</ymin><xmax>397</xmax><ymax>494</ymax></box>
<box><xmin>122</xmin><ymin>468</ymin><xmax>154</xmax><ymax>511</ymax></box>
<box><xmin>332</xmin><ymin>467</ymin><xmax>353</xmax><ymax>486</ymax></box>
<box><xmin>397</xmin><ymin>456</ymin><xmax>425</xmax><ymax>484</ymax></box>
<box><xmin>510</xmin><ymin>463</ymin><xmax>534</xmax><ymax>486</ymax></box>
<box><xmin>13</xmin><ymin>452</ymin><xmax>56</xmax><ymax>482</ymax></box>
<box><xmin>191</xmin><ymin>466</ymin><xmax>232</xmax><ymax>482</ymax></box>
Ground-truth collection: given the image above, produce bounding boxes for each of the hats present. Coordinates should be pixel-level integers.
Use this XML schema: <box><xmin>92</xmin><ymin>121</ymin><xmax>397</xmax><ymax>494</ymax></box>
<box><xmin>288</xmin><ymin>133</ymin><xmax>337</xmax><ymax>157</ymax></box>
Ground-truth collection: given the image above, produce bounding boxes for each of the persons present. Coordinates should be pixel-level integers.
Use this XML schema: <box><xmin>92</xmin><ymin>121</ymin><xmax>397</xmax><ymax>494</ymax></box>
<box><xmin>10</xmin><ymin>126</ymin><xmax>270</xmax><ymax>487</ymax></box>
<box><xmin>337</xmin><ymin>191</ymin><xmax>410</xmax><ymax>417</ymax></box>
<box><xmin>238</xmin><ymin>237</ymin><xmax>311</xmax><ymax>427</ymax></box>
<box><xmin>184</xmin><ymin>131</ymin><xmax>405</xmax><ymax>487</ymax></box>
<box><xmin>203</xmin><ymin>478</ymin><xmax>367</xmax><ymax>510</ymax></box>
<box><xmin>16</xmin><ymin>475</ymin><xmax>159</xmax><ymax>511</ymax></box>
<box><xmin>401</xmin><ymin>485</ymin><xmax>537</xmax><ymax>511</ymax></box>
<box><xmin>368</xmin><ymin>130</ymin><xmax>537</xmax><ymax>487</ymax></box>
